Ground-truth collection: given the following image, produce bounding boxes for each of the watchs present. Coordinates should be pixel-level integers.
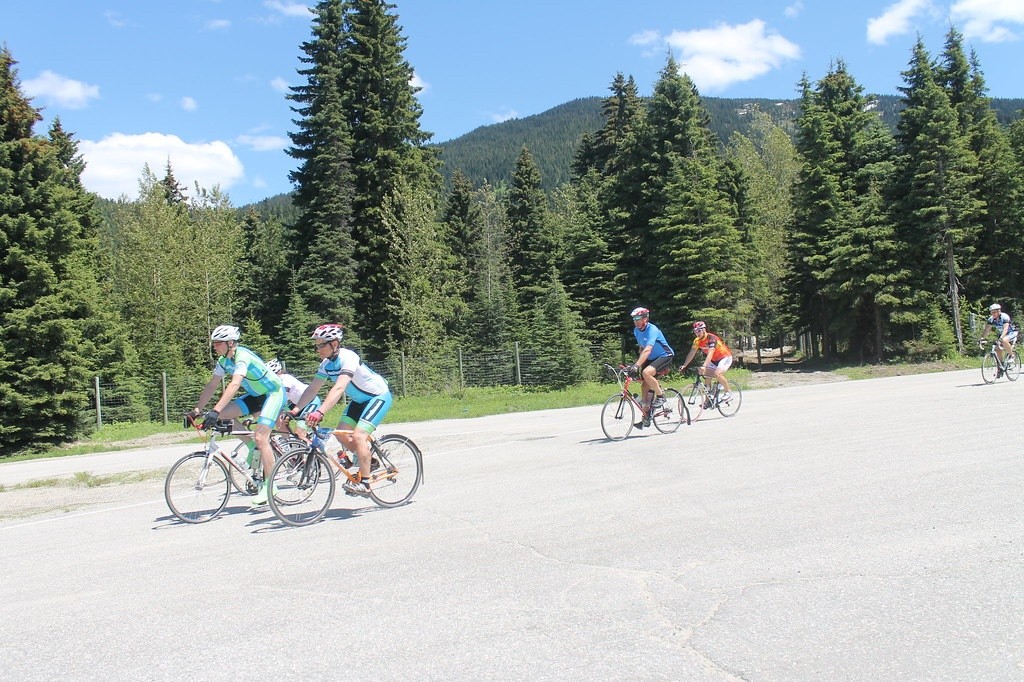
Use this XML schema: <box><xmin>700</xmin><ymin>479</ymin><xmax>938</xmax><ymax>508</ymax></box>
<box><xmin>210</xmin><ymin>409</ymin><xmax>220</xmax><ymax>415</ymax></box>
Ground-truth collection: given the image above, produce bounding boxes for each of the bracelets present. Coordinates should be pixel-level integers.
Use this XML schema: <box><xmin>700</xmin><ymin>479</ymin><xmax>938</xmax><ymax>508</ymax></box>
<box><xmin>316</xmin><ymin>409</ymin><xmax>324</xmax><ymax>416</ymax></box>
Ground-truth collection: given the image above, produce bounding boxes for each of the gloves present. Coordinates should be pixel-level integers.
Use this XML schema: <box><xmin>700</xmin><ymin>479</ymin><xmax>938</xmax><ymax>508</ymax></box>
<box><xmin>202</xmin><ymin>410</ymin><xmax>218</xmax><ymax>430</ymax></box>
<box><xmin>628</xmin><ymin>364</ymin><xmax>638</xmax><ymax>376</ymax></box>
<box><xmin>184</xmin><ymin>411</ymin><xmax>198</xmax><ymax>428</ymax></box>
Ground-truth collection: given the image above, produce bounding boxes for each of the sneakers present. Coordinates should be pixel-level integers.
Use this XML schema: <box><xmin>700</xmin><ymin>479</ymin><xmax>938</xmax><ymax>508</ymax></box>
<box><xmin>252</xmin><ymin>485</ymin><xmax>278</xmax><ymax>504</ymax></box>
<box><xmin>343</xmin><ymin>483</ymin><xmax>371</xmax><ymax>497</ymax></box>
<box><xmin>242</xmin><ymin>446</ymin><xmax>259</xmax><ymax>469</ymax></box>
<box><xmin>652</xmin><ymin>397</ymin><xmax>666</xmax><ymax>407</ymax></box>
<box><xmin>371</xmin><ymin>460</ymin><xmax>380</xmax><ymax>470</ymax></box>
<box><xmin>634</xmin><ymin>421</ymin><xmax>650</xmax><ymax>427</ymax></box>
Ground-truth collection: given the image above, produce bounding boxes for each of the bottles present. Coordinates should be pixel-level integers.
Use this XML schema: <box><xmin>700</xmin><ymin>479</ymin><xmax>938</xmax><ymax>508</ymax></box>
<box><xmin>634</xmin><ymin>393</ymin><xmax>646</xmax><ymax>408</ymax></box>
<box><xmin>250</xmin><ymin>446</ymin><xmax>261</xmax><ymax>469</ymax></box>
<box><xmin>712</xmin><ymin>381</ymin><xmax>718</xmax><ymax>396</ymax></box>
<box><xmin>646</xmin><ymin>389</ymin><xmax>654</xmax><ymax>408</ymax></box>
<box><xmin>230</xmin><ymin>451</ymin><xmax>250</xmax><ymax>471</ymax></box>
<box><xmin>337</xmin><ymin>450</ymin><xmax>353</xmax><ymax>470</ymax></box>
<box><xmin>704</xmin><ymin>385</ymin><xmax>711</xmax><ymax>398</ymax></box>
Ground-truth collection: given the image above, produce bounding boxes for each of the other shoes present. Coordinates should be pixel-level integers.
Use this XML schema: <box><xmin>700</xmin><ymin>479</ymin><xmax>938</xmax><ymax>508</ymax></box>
<box><xmin>993</xmin><ymin>372</ymin><xmax>1003</xmax><ymax>377</ymax></box>
<box><xmin>700</xmin><ymin>402</ymin><xmax>708</xmax><ymax>407</ymax></box>
<box><xmin>1008</xmin><ymin>355</ymin><xmax>1015</xmax><ymax>363</ymax></box>
<box><xmin>722</xmin><ymin>392</ymin><xmax>732</xmax><ymax>400</ymax></box>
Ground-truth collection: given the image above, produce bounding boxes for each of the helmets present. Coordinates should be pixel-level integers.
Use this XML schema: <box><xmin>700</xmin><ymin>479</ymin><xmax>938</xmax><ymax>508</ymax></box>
<box><xmin>989</xmin><ymin>303</ymin><xmax>1001</xmax><ymax>311</ymax></box>
<box><xmin>211</xmin><ymin>325</ymin><xmax>240</xmax><ymax>341</ymax></box>
<box><xmin>631</xmin><ymin>307</ymin><xmax>649</xmax><ymax>317</ymax></box>
<box><xmin>312</xmin><ymin>324</ymin><xmax>343</xmax><ymax>342</ymax></box>
<box><xmin>693</xmin><ymin>322</ymin><xmax>705</xmax><ymax>331</ymax></box>
<box><xmin>265</xmin><ymin>358</ymin><xmax>282</xmax><ymax>373</ymax></box>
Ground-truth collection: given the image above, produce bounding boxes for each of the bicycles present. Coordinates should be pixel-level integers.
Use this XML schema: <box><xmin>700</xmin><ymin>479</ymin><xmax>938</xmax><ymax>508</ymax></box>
<box><xmin>600</xmin><ymin>363</ymin><xmax>685</xmax><ymax>442</ymax></box>
<box><xmin>164</xmin><ymin>415</ymin><xmax>345</xmax><ymax>524</ymax></box>
<box><xmin>677</xmin><ymin>366</ymin><xmax>742</xmax><ymax>422</ymax></box>
<box><xmin>266</xmin><ymin>411</ymin><xmax>425</xmax><ymax>528</ymax></box>
<box><xmin>979</xmin><ymin>338</ymin><xmax>1022</xmax><ymax>384</ymax></box>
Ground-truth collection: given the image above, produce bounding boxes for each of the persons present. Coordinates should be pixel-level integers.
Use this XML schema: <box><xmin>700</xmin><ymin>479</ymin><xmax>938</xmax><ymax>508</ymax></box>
<box><xmin>983</xmin><ymin>300</ymin><xmax>1019</xmax><ymax>377</ymax></box>
<box><xmin>627</xmin><ymin>306</ymin><xmax>676</xmax><ymax>427</ymax></box>
<box><xmin>679</xmin><ymin>320</ymin><xmax>733</xmax><ymax>408</ymax></box>
<box><xmin>262</xmin><ymin>355</ymin><xmax>322</xmax><ymax>478</ymax></box>
<box><xmin>177</xmin><ymin>322</ymin><xmax>287</xmax><ymax>506</ymax></box>
<box><xmin>286</xmin><ymin>323</ymin><xmax>393</xmax><ymax>499</ymax></box>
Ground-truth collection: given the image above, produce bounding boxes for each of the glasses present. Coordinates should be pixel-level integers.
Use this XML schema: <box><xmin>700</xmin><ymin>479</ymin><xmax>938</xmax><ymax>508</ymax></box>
<box><xmin>633</xmin><ymin>316</ymin><xmax>644</xmax><ymax>320</ymax></box>
<box><xmin>213</xmin><ymin>341</ymin><xmax>225</xmax><ymax>346</ymax></box>
<box><xmin>316</xmin><ymin>341</ymin><xmax>332</xmax><ymax>348</ymax></box>
